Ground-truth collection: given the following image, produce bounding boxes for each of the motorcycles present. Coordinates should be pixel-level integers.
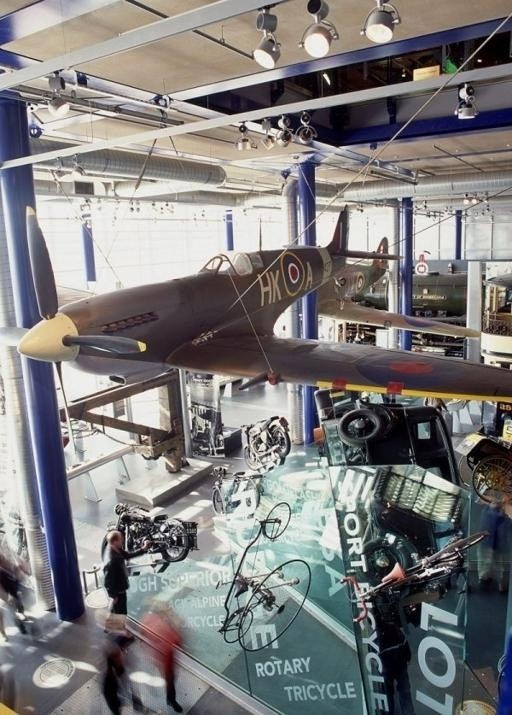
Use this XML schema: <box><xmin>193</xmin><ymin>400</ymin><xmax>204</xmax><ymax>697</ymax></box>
<box><xmin>209</xmin><ymin>467</ymin><xmax>260</xmax><ymax>516</ymax></box>
<box><xmin>101</xmin><ymin>503</ymin><xmax>199</xmax><ymax>563</ymax></box>
<box><xmin>240</xmin><ymin>415</ymin><xmax>290</xmax><ymax>471</ymax></box>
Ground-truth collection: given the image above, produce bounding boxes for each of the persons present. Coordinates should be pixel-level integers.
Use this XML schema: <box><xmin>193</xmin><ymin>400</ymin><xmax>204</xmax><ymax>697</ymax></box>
<box><xmin>0</xmin><ymin>547</ymin><xmax>26</xmax><ymax>642</ymax></box>
<box><xmin>100</xmin><ymin>530</ymin><xmax>153</xmax><ymax>634</ymax></box>
<box><xmin>138</xmin><ymin>599</ymin><xmax>187</xmax><ymax>712</ymax></box>
<box><xmin>192</xmin><ymin>416</ymin><xmax>198</xmax><ymax>438</ymax></box>
<box><xmin>204</xmin><ymin>423</ymin><xmax>217</xmax><ymax>456</ymax></box>
<box><xmin>100</xmin><ymin>630</ymin><xmax>146</xmax><ymax>715</ymax></box>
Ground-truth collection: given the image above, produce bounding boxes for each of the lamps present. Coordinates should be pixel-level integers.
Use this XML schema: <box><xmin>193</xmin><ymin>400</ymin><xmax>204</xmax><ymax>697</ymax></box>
<box><xmin>236</xmin><ymin>111</ymin><xmax>317</xmax><ymax>152</ymax></box>
<box><xmin>252</xmin><ymin>0</ymin><xmax>402</xmax><ymax>70</ymax></box>
<box><xmin>48</xmin><ymin>71</ymin><xmax>70</xmax><ymax>119</ymax></box>
<box><xmin>54</xmin><ymin>153</ymin><xmax>86</xmax><ymax>181</ymax></box>
<box><xmin>453</xmin><ymin>81</ymin><xmax>481</xmax><ymax>121</ymax></box>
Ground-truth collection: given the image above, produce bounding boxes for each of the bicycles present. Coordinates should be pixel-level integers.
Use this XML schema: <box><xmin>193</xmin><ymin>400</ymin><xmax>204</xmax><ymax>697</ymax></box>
<box><xmin>338</xmin><ymin>532</ymin><xmax>488</xmax><ymax>626</ymax></box>
